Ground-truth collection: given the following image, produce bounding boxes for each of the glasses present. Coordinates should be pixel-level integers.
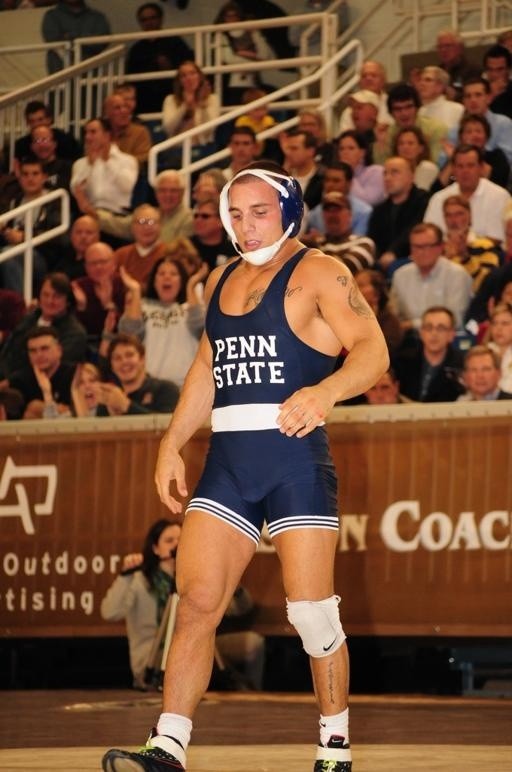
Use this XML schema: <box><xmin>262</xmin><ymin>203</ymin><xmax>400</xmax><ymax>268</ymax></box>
<box><xmin>194</xmin><ymin>213</ymin><xmax>216</xmax><ymax>219</ymax></box>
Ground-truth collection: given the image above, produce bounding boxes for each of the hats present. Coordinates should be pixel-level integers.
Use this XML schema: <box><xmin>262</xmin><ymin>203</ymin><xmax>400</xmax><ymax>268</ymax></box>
<box><xmin>322</xmin><ymin>191</ymin><xmax>350</xmax><ymax>209</ymax></box>
<box><xmin>345</xmin><ymin>89</ymin><xmax>379</xmax><ymax>111</ymax></box>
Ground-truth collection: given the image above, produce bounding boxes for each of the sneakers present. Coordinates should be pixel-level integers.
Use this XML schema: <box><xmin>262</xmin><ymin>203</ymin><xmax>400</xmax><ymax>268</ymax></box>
<box><xmin>314</xmin><ymin>735</ymin><xmax>352</xmax><ymax>772</ymax></box>
<box><xmin>102</xmin><ymin>727</ymin><xmax>186</xmax><ymax>772</ymax></box>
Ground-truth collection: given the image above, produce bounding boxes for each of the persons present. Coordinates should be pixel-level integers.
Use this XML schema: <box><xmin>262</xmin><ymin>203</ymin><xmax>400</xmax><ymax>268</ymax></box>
<box><xmin>2</xmin><ymin>1</ymin><xmax>512</xmax><ymax>421</ymax></box>
<box><xmin>102</xmin><ymin>518</ymin><xmax>265</xmax><ymax>692</ymax></box>
<box><xmin>103</xmin><ymin>162</ymin><xmax>389</xmax><ymax>772</ymax></box>
